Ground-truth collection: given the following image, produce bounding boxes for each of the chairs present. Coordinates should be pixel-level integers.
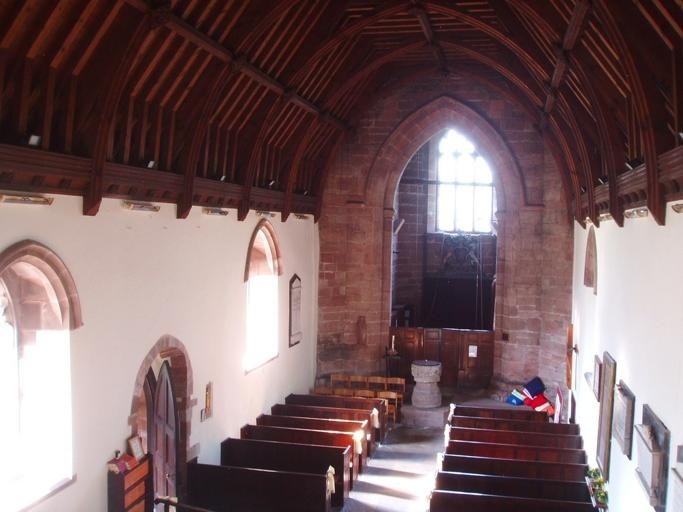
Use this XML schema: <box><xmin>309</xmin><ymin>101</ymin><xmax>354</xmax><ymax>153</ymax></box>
<box><xmin>431</xmin><ymin>403</ymin><xmax>600</xmax><ymax>511</ymax></box>
<box><xmin>314</xmin><ymin>373</ymin><xmax>406</xmax><ymax>424</ymax></box>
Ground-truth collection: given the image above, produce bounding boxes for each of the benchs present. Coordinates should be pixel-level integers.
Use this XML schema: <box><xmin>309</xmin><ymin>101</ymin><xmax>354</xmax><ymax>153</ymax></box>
<box><xmin>185</xmin><ymin>393</ymin><xmax>387</xmax><ymax>512</ymax></box>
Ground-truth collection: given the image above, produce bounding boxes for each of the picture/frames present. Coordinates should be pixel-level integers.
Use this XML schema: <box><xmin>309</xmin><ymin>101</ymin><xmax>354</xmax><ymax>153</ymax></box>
<box><xmin>289</xmin><ymin>273</ymin><xmax>302</xmax><ymax>347</ymax></box>
<box><xmin>128</xmin><ymin>435</ymin><xmax>145</xmax><ymax>463</ymax></box>
<box><xmin>590</xmin><ymin>350</ymin><xmax>670</xmax><ymax>512</ymax></box>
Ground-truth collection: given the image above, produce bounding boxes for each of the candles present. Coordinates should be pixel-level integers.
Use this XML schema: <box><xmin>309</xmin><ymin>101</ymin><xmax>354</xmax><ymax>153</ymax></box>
<box><xmin>391</xmin><ymin>334</ymin><xmax>396</xmax><ymax>352</ymax></box>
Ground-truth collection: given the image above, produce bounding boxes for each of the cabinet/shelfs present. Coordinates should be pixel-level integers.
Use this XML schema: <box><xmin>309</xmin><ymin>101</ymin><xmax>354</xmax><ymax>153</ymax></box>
<box><xmin>108</xmin><ymin>452</ymin><xmax>154</xmax><ymax>512</ymax></box>
